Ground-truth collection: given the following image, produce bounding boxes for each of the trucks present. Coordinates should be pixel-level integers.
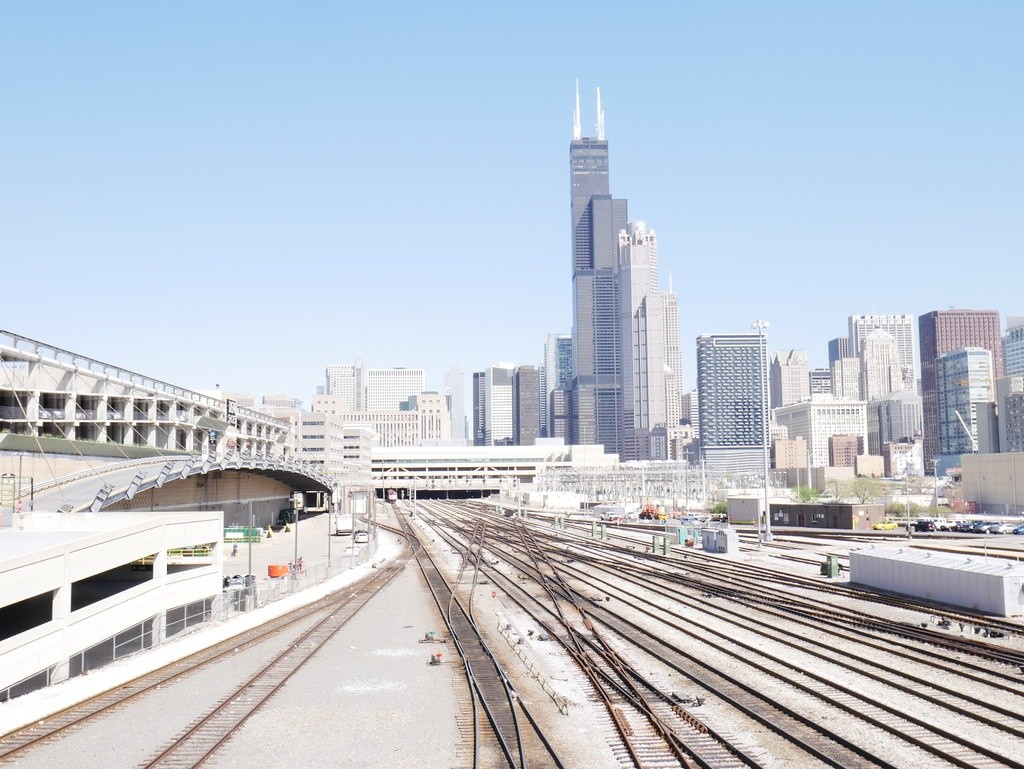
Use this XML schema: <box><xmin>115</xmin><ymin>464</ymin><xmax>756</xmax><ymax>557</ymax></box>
<box><xmin>335</xmin><ymin>514</ymin><xmax>354</xmax><ymax>535</ymax></box>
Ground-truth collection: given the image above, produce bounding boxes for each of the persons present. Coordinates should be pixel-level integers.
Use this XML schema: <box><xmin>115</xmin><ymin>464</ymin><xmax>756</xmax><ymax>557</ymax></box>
<box><xmin>232</xmin><ymin>542</ymin><xmax>238</xmax><ymax>557</ymax></box>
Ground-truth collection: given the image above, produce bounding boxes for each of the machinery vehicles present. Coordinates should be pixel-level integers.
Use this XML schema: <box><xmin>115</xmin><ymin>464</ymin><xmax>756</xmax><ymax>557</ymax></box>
<box><xmin>640</xmin><ymin>504</ymin><xmax>660</xmax><ymax>520</ymax></box>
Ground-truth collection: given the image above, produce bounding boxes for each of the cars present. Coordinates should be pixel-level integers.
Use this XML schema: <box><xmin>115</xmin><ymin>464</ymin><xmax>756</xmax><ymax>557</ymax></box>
<box><xmin>624</xmin><ymin>512</ymin><xmax>639</xmax><ymax>519</ymax></box>
<box><xmin>872</xmin><ymin>519</ymin><xmax>897</xmax><ymax>531</ymax></box>
<box><xmin>906</xmin><ymin>518</ymin><xmax>1024</xmax><ymax>534</ymax></box>
<box><xmin>344</xmin><ymin>545</ymin><xmax>361</xmax><ymax>555</ymax></box>
<box><xmin>354</xmin><ymin>533</ymin><xmax>368</xmax><ymax>543</ymax></box>
<box><xmin>712</xmin><ymin>514</ymin><xmax>728</xmax><ymax>522</ymax></box>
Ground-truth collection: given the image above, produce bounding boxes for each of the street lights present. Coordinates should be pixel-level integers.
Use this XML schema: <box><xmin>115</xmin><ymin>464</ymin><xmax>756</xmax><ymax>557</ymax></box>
<box><xmin>381</xmin><ymin>460</ymin><xmax>385</xmax><ymax>504</ymax></box>
<box><xmin>931</xmin><ymin>458</ymin><xmax>941</xmax><ymax>512</ymax></box>
<box><xmin>751</xmin><ymin>319</ymin><xmax>776</xmax><ymax>542</ymax></box>
<box><xmin>238</xmin><ymin>501</ymin><xmax>251</xmax><ymax>588</ymax></box>
<box><xmin>289</xmin><ymin>498</ymin><xmax>298</xmax><ymax>573</ymax></box>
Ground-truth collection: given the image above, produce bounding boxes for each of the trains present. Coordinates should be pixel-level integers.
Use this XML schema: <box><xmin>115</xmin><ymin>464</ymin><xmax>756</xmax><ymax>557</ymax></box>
<box><xmin>385</xmin><ymin>489</ymin><xmax>397</xmax><ymax>502</ymax></box>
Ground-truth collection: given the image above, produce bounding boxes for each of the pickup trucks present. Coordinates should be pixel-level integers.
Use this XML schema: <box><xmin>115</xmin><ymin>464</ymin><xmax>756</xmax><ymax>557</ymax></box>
<box><xmin>682</xmin><ymin>513</ymin><xmax>702</xmax><ymax>521</ymax></box>
<box><xmin>600</xmin><ymin>512</ymin><xmax>619</xmax><ymax>520</ymax></box>
